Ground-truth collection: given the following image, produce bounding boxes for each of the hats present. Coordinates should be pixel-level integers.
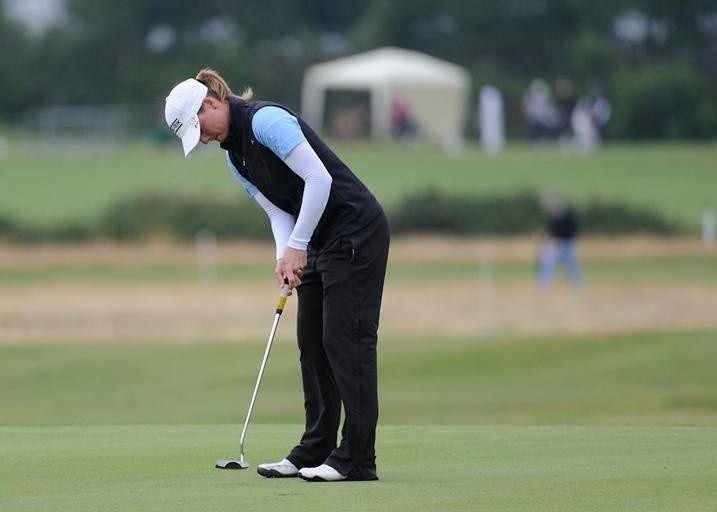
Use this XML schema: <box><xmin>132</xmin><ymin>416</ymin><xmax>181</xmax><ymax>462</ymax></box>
<box><xmin>165</xmin><ymin>78</ymin><xmax>208</xmax><ymax>158</ymax></box>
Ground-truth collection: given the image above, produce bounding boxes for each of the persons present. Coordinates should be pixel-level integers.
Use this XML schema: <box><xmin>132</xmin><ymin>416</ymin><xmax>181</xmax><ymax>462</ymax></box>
<box><xmin>164</xmin><ymin>66</ymin><xmax>391</xmax><ymax>482</ymax></box>
<box><xmin>538</xmin><ymin>191</ymin><xmax>583</xmax><ymax>283</ymax></box>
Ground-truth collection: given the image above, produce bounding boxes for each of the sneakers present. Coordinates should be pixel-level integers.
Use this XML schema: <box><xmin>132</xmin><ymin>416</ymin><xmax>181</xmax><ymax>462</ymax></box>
<box><xmin>257</xmin><ymin>458</ymin><xmax>348</xmax><ymax>481</ymax></box>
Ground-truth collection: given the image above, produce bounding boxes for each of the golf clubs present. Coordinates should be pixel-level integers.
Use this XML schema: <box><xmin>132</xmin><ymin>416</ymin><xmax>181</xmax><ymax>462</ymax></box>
<box><xmin>216</xmin><ymin>277</ymin><xmax>289</xmax><ymax>470</ymax></box>
